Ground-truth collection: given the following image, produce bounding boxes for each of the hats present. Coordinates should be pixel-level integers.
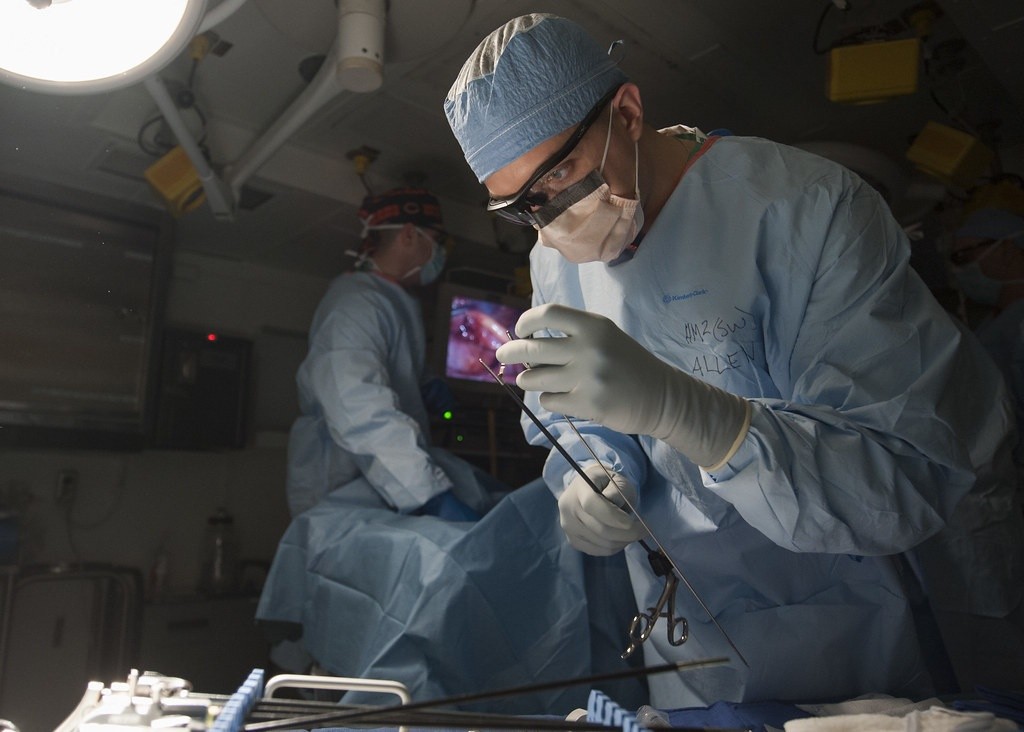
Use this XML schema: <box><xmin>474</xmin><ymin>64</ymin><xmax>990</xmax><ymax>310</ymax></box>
<box><xmin>441</xmin><ymin>11</ymin><xmax>632</xmax><ymax>184</ymax></box>
<box><xmin>358</xmin><ymin>184</ymin><xmax>444</xmax><ymax>228</ymax></box>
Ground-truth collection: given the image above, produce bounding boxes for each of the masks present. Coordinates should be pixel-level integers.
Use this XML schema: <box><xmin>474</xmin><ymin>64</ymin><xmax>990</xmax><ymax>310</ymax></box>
<box><xmin>537</xmin><ymin>100</ymin><xmax>645</xmax><ymax>264</ymax></box>
<box><xmin>344</xmin><ymin>224</ymin><xmax>449</xmax><ymax>286</ymax></box>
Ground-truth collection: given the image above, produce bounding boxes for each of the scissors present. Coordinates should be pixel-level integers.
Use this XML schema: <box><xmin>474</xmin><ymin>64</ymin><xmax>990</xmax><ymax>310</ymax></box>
<box><xmin>477</xmin><ymin>330</ymin><xmax>696</xmax><ymax>663</ymax></box>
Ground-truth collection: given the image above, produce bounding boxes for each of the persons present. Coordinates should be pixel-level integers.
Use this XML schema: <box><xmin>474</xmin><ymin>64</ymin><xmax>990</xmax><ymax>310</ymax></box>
<box><xmin>444</xmin><ymin>12</ymin><xmax>1017</xmax><ymax>712</ymax></box>
<box><xmin>286</xmin><ymin>185</ymin><xmax>483</xmax><ymax>522</ymax></box>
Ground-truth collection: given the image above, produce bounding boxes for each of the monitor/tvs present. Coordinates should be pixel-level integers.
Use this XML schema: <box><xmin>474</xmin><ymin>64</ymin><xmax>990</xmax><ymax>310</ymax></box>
<box><xmin>430</xmin><ymin>282</ymin><xmax>532</xmax><ymax>396</ymax></box>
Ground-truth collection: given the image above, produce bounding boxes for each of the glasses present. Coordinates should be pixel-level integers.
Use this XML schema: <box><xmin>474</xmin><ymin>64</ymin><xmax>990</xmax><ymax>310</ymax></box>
<box><xmin>485</xmin><ymin>80</ymin><xmax>625</xmax><ymax>227</ymax></box>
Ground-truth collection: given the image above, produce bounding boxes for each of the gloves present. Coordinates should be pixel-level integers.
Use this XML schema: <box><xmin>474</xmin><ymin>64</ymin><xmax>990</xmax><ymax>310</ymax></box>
<box><xmin>422</xmin><ymin>491</ymin><xmax>482</xmax><ymax>522</ymax></box>
<box><xmin>557</xmin><ymin>458</ymin><xmax>653</xmax><ymax>557</ymax></box>
<box><xmin>495</xmin><ymin>304</ymin><xmax>752</xmax><ymax>473</ymax></box>
